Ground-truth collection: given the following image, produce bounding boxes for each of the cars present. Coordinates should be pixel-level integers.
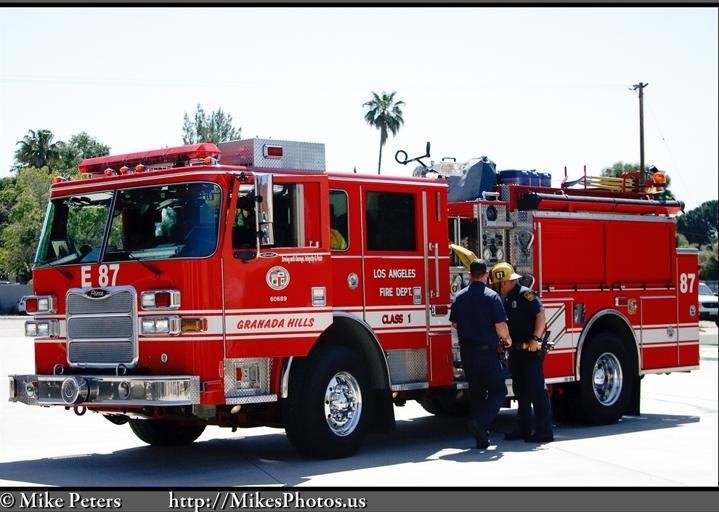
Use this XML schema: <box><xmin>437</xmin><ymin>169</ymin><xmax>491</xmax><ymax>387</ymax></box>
<box><xmin>697</xmin><ymin>283</ymin><xmax>718</xmax><ymax>321</ymax></box>
<box><xmin>17</xmin><ymin>295</ymin><xmax>34</xmax><ymax>316</ymax></box>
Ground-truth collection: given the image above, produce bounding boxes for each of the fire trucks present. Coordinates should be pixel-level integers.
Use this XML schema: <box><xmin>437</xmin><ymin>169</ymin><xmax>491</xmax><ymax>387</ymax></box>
<box><xmin>8</xmin><ymin>139</ymin><xmax>699</xmax><ymax>460</ymax></box>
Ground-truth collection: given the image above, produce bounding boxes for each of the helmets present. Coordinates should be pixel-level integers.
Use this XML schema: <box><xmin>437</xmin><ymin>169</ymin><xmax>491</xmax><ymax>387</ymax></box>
<box><xmin>490</xmin><ymin>261</ymin><xmax>522</xmax><ymax>283</ymax></box>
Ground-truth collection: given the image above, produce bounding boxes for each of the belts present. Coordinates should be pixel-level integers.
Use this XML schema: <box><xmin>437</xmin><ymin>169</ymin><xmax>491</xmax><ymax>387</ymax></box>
<box><xmin>460</xmin><ymin>344</ymin><xmax>493</xmax><ymax>351</ymax></box>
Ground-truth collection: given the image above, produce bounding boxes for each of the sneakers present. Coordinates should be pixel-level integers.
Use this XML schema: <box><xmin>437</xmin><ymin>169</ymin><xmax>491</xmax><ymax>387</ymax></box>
<box><xmin>524</xmin><ymin>431</ymin><xmax>554</xmax><ymax>443</ymax></box>
<box><xmin>504</xmin><ymin>427</ymin><xmax>532</xmax><ymax>441</ymax></box>
<box><xmin>474</xmin><ymin>431</ymin><xmax>491</xmax><ymax>449</ymax></box>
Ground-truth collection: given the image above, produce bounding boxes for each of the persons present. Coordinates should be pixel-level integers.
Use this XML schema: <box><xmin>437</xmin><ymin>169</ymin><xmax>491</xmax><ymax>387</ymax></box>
<box><xmin>448</xmin><ymin>257</ymin><xmax>513</xmax><ymax>449</ymax></box>
<box><xmin>486</xmin><ymin>261</ymin><xmax>556</xmax><ymax>443</ymax></box>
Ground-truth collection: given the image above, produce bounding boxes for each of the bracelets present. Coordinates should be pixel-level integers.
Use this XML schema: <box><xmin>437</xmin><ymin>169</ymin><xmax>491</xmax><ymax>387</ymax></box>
<box><xmin>531</xmin><ymin>335</ymin><xmax>539</xmax><ymax>340</ymax></box>
